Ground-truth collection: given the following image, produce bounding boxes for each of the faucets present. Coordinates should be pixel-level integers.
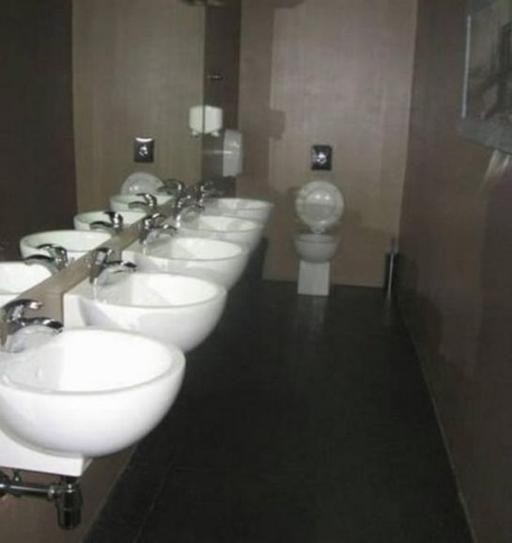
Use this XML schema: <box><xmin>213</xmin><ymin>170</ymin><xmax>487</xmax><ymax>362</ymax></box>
<box><xmin>90</xmin><ymin>210</ymin><xmax>124</xmax><ymax>230</ymax></box>
<box><xmin>127</xmin><ymin>192</ymin><xmax>158</xmax><ymax>212</ymax></box>
<box><xmin>4</xmin><ymin>300</ymin><xmax>63</xmax><ymax>347</ymax></box>
<box><xmin>173</xmin><ymin>195</ymin><xmax>206</xmax><ymax>222</ymax></box>
<box><xmin>139</xmin><ymin>215</ymin><xmax>178</xmax><ymax>244</ymax></box>
<box><xmin>88</xmin><ymin>249</ymin><xmax>137</xmax><ymax>285</ymax></box>
<box><xmin>23</xmin><ymin>244</ymin><xmax>68</xmax><ymax>273</ymax></box>
<box><xmin>192</xmin><ymin>178</ymin><xmax>226</xmax><ymax>209</ymax></box>
<box><xmin>156</xmin><ymin>178</ymin><xmax>184</xmax><ymax>195</ymax></box>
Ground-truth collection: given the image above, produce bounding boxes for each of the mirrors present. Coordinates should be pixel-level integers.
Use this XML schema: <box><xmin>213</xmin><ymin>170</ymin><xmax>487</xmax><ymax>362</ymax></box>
<box><xmin>0</xmin><ymin>0</ymin><xmax>205</xmax><ymax>308</ymax></box>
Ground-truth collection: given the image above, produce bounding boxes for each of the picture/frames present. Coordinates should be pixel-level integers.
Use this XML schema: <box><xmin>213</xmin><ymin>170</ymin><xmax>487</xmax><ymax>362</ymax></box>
<box><xmin>453</xmin><ymin>0</ymin><xmax>511</xmax><ymax>153</ymax></box>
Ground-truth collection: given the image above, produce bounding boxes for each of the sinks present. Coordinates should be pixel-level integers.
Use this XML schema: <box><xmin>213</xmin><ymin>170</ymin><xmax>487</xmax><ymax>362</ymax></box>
<box><xmin>142</xmin><ymin>237</ymin><xmax>250</xmax><ymax>290</ymax></box>
<box><xmin>204</xmin><ymin>197</ymin><xmax>271</xmax><ymax>222</ymax></box>
<box><xmin>0</xmin><ymin>260</ymin><xmax>56</xmax><ymax>308</ymax></box>
<box><xmin>1</xmin><ymin>324</ymin><xmax>186</xmax><ymax>476</ymax></box>
<box><xmin>74</xmin><ymin>207</ymin><xmax>144</xmax><ymax>230</ymax></box>
<box><xmin>20</xmin><ymin>228</ymin><xmax>112</xmax><ymax>257</ymax></box>
<box><xmin>179</xmin><ymin>210</ymin><xmax>264</xmax><ymax>250</ymax></box>
<box><xmin>109</xmin><ymin>189</ymin><xmax>172</xmax><ymax>211</ymax></box>
<box><xmin>82</xmin><ymin>270</ymin><xmax>227</xmax><ymax>351</ymax></box>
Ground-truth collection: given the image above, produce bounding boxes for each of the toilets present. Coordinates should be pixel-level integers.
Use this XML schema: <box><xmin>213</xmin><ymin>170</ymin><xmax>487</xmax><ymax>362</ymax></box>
<box><xmin>292</xmin><ymin>179</ymin><xmax>345</xmax><ymax>297</ymax></box>
<box><xmin>118</xmin><ymin>171</ymin><xmax>163</xmax><ymax>191</ymax></box>
